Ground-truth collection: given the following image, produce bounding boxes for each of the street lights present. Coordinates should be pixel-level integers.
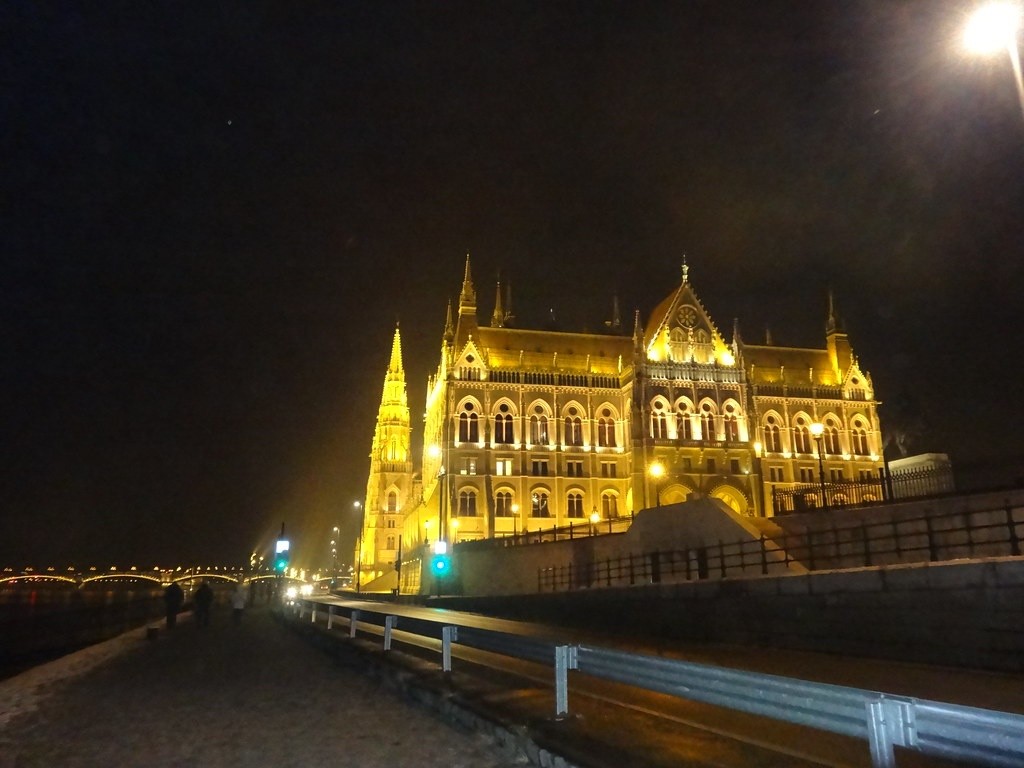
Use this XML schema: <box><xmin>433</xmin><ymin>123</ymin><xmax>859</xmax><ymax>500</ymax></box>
<box><xmin>591</xmin><ymin>505</ymin><xmax>598</xmax><ymax>535</ymax></box>
<box><xmin>512</xmin><ymin>503</ymin><xmax>520</xmax><ymax>537</ymax></box>
<box><xmin>354</xmin><ymin>501</ymin><xmax>362</xmax><ymax>593</ymax></box>
<box><xmin>650</xmin><ymin>461</ymin><xmax>661</xmax><ymax>506</ymax></box>
<box><xmin>330</xmin><ymin>526</ymin><xmax>340</xmax><ymax>591</ymax></box>
<box><xmin>810</xmin><ymin>420</ymin><xmax>830</xmax><ymax>508</ymax></box>
<box><xmin>424</xmin><ymin>520</ymin><xmax>430</xmax><ymax>546</ymax></box>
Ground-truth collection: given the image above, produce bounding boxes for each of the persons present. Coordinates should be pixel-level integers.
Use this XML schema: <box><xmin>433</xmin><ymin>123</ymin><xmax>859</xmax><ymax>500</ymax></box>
<box><xmin>231</xmin><ymin>584</ymin><xmax>244</xmax><ymax>624</ymax></box>
<box><xmin>192</xmin><ymin>583</ymin><xmax>213</xmax><ymax>630</ymax></box>
<box><xmin>163</xmin><ymin>581</ymin><xmax>184</xmax><ymax>629</ymax></box>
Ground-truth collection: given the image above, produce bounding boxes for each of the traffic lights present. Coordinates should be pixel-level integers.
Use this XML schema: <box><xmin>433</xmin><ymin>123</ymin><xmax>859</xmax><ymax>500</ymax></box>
<box><xmin>434</xmin><ymin>557</ymin><xmax>445</xmax><ymax>571</ymax></box>
<box><xmin>276</xmin><ymin>557</ymin><xmax>285</xmax><ymax>571</ymax></box>
